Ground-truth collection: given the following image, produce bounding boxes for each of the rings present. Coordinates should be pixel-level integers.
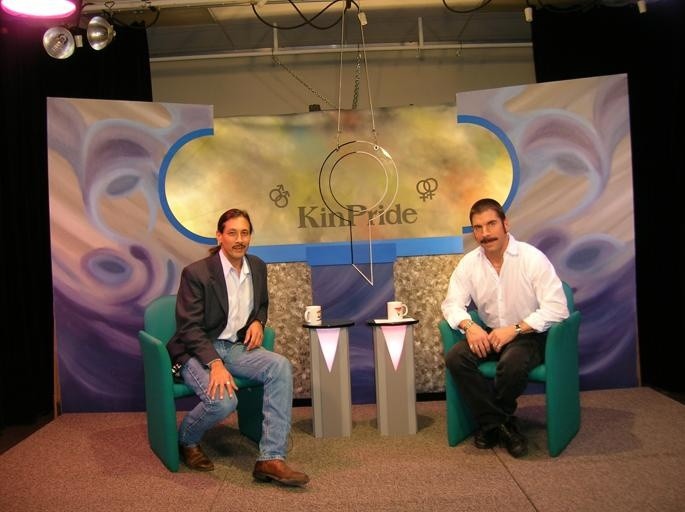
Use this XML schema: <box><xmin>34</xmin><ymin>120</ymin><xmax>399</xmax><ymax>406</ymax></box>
<box><xmin>226</xmin><ymin>381</ymin><xmax>231</xmax><ymax>384</ymax></box>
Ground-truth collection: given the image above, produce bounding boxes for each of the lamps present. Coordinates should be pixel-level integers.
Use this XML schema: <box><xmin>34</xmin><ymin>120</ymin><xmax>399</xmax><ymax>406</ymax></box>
<box><xmin>42</xmin><ymin>16</ymin><xmax>117</xmax><ymax>59</ymax></box>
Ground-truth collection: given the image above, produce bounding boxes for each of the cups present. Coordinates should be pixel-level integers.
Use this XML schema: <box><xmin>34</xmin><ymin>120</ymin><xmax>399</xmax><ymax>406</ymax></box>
<box><xmin>304</xmin><ymin>305</ymin><xmax>322</xmax><ymax>325</ymax></box>
<box><xmin>387</xmin><ymin>302</ymin><xmax>409</xmax><ymax>322</ymax></box>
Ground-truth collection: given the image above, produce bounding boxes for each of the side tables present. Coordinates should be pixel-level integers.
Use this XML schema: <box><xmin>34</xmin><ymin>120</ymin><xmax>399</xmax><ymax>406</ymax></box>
<box><xmin>302</xmin><ymin>319</ymin><xmax>355</xmax><ymax>438</ymax></box>
<box><xmin>365</xmin><ymin>315</ymin><xmax>420</xmax><ymax>436</ymax></box>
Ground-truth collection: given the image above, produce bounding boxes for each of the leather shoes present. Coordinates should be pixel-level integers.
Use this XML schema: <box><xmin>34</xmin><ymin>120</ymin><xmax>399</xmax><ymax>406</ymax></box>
<box><xmin>252</xmin><ymin>458</ymin><xmax>310</xmax><ymax>488</ymax></box>
<box><xmin>475</xmin><ymin>421</ymin><xmax>498</xmax><ymax>450</ymax></box>
<box><xmin>498</xmin><ymin>414</ymin><xmax>530</xmax><ymax>459</ymax></box>
<box><xmin>178</xmin><ymin>444</ymin><xmax>215</xmax><ymax>472</ymax></box>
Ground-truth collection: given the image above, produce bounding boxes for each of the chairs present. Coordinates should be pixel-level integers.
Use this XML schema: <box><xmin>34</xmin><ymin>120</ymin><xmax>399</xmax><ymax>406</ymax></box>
<box><xmin>438</xmin><ymin>279</ymin><xmax>580</xmax><ymax>457</ymax></box>
<box><xmin>138</xmin><ymin>294</ymin><xmax>275</xmax><ymax>473</ymax></box>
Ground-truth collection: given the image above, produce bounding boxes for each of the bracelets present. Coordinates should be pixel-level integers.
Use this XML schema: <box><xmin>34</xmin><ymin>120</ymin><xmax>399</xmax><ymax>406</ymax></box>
<box><xmin>463</xmin><ymin>320</ymin><xmax>474</xmax><ymax>331</ymax></box>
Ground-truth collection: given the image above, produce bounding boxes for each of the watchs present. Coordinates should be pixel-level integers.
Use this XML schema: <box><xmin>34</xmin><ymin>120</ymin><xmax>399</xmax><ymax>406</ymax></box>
<box><xmin>515</xmin><ymin>325</ymin><xmax>522</xmax><ymax>335</ymax></box>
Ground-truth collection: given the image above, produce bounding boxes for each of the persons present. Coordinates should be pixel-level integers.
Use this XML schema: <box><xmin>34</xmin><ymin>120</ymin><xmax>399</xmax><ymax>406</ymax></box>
<box><xmin>165</xmin><ymin>209</ymin><xmax>310</xmax><ymax>488</ymax></box>
<box><xmin>440</xmin><ymin>199</ymin><xmax>570</xmax><ymax>457</ymax></box>
